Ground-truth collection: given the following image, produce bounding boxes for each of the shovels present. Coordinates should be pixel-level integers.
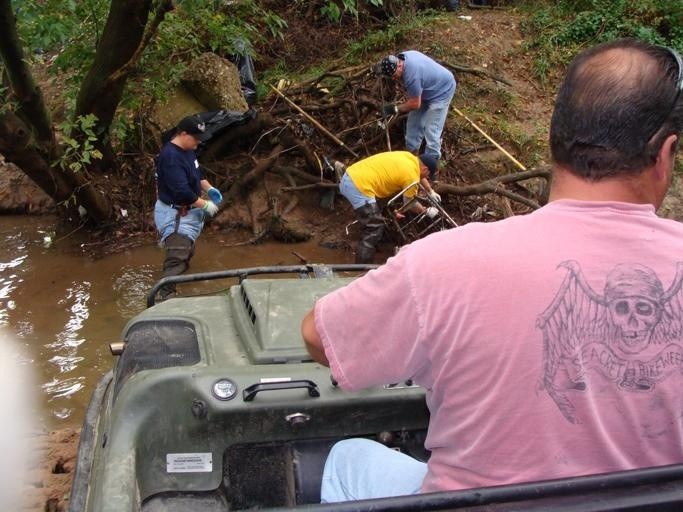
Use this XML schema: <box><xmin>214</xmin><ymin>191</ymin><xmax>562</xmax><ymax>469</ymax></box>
<box><xmin>452</xmin><ymin>107</ymin><xmax>545</xmax><ymax>194</ymax></box>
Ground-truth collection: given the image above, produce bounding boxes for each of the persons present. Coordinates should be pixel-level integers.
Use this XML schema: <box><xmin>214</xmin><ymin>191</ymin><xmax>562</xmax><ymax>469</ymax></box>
<box><xmin>301</xmin><ymin>38</ymin><xmax>683</xmax><ymax>506</ymax></box>
<box><xmin>154</xmin><ymin>115</ymin><xmax>222</xmax><ymax>300</ymax></box>
<box><xmin>339</xmin><ymin>151</ymin><xmax>441</xmax><ymax>264</ymax></box>
<box><xmin>375</xmin><ymin>50</ymin><xmax>457</xmax><ymax>159</ymax></box>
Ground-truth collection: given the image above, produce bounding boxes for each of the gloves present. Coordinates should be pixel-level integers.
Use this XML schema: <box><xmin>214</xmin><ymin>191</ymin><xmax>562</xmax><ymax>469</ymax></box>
<box><xmin>425</xmin><ymin>206</ymin><xmax>439</xmax><ymax>219</ymax></box>
<box><xmin>430</xmin><ymin>189</ymin><xmax>442</xmax><ymax>202</ymax></box>
<box><xmin>202</xmin><ymin>200</ymin><xmax>219</xmax><ymax>217</ymax></box>
<box><xmin>384</xmin><ymin>105</ymin><xmax>398</xmax><ymax>114</ymax></box>
<box><xmin>208</xmin><ymin>187</ymin><xmax>223</xmax><ymax>204</ymax></box>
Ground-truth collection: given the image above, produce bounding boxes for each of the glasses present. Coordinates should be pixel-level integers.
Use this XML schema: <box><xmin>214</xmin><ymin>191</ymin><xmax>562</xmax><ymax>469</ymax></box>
<box><xmin>644</xmin><ymin>45</ymin><xmax>683</xmax><ymax>147</ymax></box>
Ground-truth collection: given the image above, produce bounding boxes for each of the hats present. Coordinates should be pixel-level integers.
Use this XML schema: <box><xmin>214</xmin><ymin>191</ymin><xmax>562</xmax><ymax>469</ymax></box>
<box><xmin>420</xmin><ymin>153</ymin><xmax>436</xmax><ymax>181</ymax></box>
<box><xmin>381</xmin><ymin>55</ymin><xmax>399</xmax><ymax>76</ymax></box>
<box><xmin>179</xmin><ymin>116</ymin><xmax>214</xmax><ymax>142</ymax></box>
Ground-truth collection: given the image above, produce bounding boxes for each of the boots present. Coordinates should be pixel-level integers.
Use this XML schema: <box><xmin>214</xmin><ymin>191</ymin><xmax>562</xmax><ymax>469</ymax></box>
<box><xmin>159</xmin><ymin>234</ymin><xmax>194</xmax><ymax>297</ymax></box>
<box><xmin>355</xmin><ymin>203</ymin><xmax>385</xmax><ymax>265</ymax></box>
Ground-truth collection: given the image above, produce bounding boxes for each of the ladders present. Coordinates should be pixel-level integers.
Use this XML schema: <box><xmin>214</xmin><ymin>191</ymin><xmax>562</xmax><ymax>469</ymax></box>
<box><xmin>386</xmin><ymin>180</ymin><xmax>460</xmax><ymax>245</ymax></box>
